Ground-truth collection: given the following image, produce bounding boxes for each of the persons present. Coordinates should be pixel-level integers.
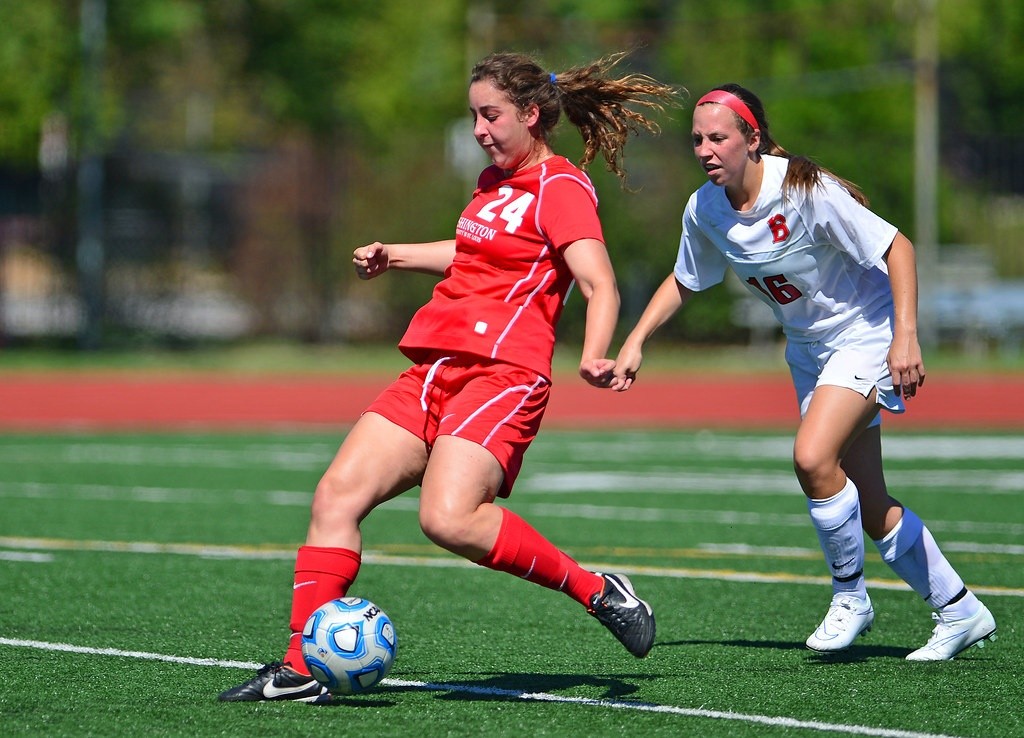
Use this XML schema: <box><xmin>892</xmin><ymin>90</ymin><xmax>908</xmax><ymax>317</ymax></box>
<box><xmin>214</xmin><ymin>52</ymin><xmax>691</xmax><ymax>702</ymax></box>
<box><xmin>613</xmin><ymin>84</ymin><xmax>997</xmax><ymax>661</ymax></box>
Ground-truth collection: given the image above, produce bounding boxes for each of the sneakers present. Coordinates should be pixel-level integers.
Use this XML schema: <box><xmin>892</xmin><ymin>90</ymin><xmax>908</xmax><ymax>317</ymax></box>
<box><xmin>806</xmin><ymin>593</ymin><xmax>875</xmax><ymax>654</ymax></box>
<box><xmin>905</xmin><ymin>602</ymin><xmax>998</xmax><ymax>662</ymax></box>
<box><xmin>586</xmin><ymin>572</ymin><xmax>655</xmax><ymax>658</ymax></box>
<box><xmin>219</xmin><ymin>661</ymin><xmax>332</xmax><ymax>704</ymax></box>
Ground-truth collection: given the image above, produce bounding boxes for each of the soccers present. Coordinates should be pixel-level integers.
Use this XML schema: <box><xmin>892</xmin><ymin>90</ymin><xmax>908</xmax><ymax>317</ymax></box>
<box><xmin>299</xmin><ymin>595</ymin><xmax>398</xmax><ymax>693</ymax></box>
